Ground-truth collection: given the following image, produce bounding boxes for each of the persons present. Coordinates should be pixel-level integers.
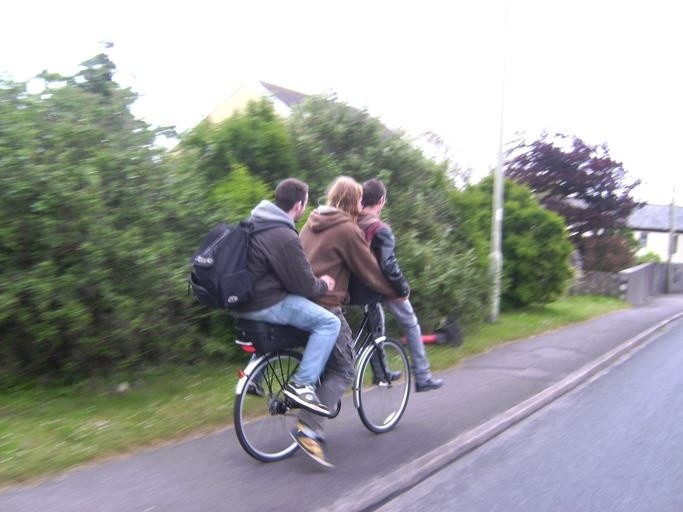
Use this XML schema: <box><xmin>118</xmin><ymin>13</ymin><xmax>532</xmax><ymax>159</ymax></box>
<box><xmin>228</xmin><ymin>179</ymin><xmax>341</xmax><ymax>417</ymax></box>
<box><xmin>341</xmin><ymin>179</ymin><xmax>443</xmax><ymax>390</ymax></box>
<box><xmin>288</xmin><ymin>174</ymin><xmax>402</xmax><ymax>470</ymax></box>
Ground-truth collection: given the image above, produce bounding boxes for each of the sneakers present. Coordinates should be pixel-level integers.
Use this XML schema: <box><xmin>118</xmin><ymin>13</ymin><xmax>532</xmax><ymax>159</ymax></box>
<box><xmin>289</xmin><ymin>422</ymin><xmax>334</xmax><ymax>468</ymax></box>
<box><xmin>247</xmin><ymin>380</ymin><xmax>265</xmax><ymax>398</ymax></box>
<box><xmin>283</xmin><ymin>377</ymin><xmax>330</xmax><ymax>417</ymax></box>
<box><xmin>373</xmin><ymin>371</ymin><xmax>401</xmax><ymax>384</ymax></box>
<box><xmin>416</xmin><ymin>379</ymin><xmax>443</xmax><ymax>391</ymax></box>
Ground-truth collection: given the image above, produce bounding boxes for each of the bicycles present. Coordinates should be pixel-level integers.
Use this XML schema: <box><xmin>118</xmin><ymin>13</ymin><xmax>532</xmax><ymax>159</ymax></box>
<box><xmin>232</xmin><ymin>289</ymin><xmax>415</xmax><ymax>465</ymax></box>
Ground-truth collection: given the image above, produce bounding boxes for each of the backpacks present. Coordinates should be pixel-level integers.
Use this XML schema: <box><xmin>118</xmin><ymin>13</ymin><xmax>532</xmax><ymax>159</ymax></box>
<box><xmin>190</xmin><ymin>216</ymin><xmax>299</xmax><ymax>310</ymax></box>
